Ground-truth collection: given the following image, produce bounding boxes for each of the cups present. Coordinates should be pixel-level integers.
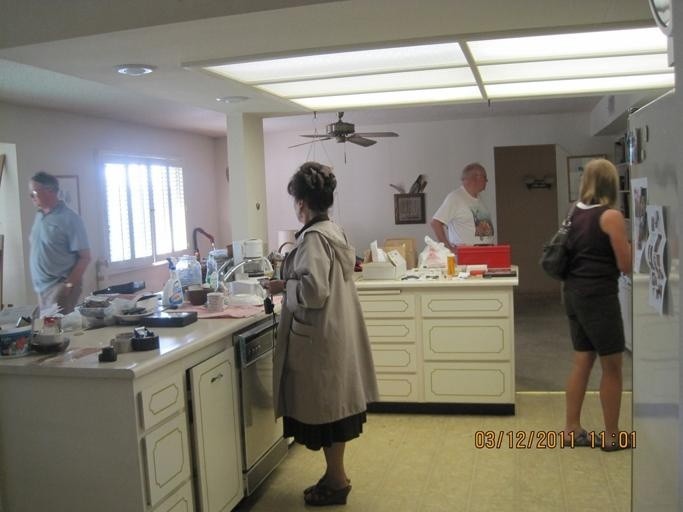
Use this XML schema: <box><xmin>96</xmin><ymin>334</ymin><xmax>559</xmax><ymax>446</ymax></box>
<box><xmin>207</xmin><ymin>292</ymin><xmax>230</xmax><ymax>313</ymax></box>
<box><xmin>31</xmin><ymin>329</ymin><xmax>64</xmax><ymax>346</ymax></box>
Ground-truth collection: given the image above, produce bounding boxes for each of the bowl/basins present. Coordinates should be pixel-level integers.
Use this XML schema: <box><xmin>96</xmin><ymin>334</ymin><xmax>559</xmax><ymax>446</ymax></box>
<box><xmin>184</xmin><ymin>290</ymin><xmax>207</xmax><ymax>305</ymax></box>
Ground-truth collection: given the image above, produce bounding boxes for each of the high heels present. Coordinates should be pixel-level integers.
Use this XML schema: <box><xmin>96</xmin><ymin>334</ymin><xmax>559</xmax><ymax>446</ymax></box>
<box><xmin>304</xmin><ymin>474</ymin><xmax>352</xmax><ymax>505</ymax></box>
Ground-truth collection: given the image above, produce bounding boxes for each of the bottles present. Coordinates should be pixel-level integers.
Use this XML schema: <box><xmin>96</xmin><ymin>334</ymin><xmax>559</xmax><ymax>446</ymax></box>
<box><xmin>206</xmin><ymin>254</ymin><xmax>218</xmax><ymax>291</ymax></box>
<box><xmin>447</xmin><ymin>255</ymin><xmax>455</xmax><ymax>275</ymax></box>
<box><xmin>176</xmin><ymin>255</ymin><xmax>202</xmax><ymax>288</ymax></box>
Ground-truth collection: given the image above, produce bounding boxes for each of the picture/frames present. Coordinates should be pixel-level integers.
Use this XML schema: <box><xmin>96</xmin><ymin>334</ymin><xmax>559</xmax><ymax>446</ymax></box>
<box><xmin>54</xmin><ymin>175</ymin><xmax>81</xmax><ymax>216</ymax></box>
<box><xmin>566</xmin><ymin>154</ymin><xmax>607</xmax><ymax>203</ymax></box>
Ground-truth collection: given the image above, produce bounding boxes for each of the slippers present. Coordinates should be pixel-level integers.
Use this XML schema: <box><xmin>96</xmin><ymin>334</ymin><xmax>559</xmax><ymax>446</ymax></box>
<box><xmin>563</xmin><ymin>430</ymin><xmax>601</xmax><ymax>446</ymax></box>
<box><xmin>601</xmin><ymin>431</ymin><xmax>632</xmax><ymax>451</ymax></box>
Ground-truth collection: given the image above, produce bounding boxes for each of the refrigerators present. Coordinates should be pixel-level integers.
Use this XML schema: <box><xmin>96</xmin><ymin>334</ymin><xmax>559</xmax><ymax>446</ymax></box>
<box><xmin>628</xmin><ymin>88</ymin><xmax>683</xmax><ymax>512</ymax></box>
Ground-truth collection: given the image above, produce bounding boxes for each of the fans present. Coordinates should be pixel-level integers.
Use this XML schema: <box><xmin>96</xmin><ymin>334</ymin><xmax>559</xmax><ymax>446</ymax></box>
<box><xmin>287</xmin><ymin>110</ymin><xmax>400</xmax><ymax>166</ymax></box>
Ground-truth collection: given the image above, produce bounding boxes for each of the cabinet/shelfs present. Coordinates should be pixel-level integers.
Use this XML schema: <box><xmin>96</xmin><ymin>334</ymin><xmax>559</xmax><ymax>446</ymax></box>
<box><xmin>614</xmin><ymin>163</ymin><xmax>629</xmax><ymax>222</ymax></box>
<box><xmin>354</xmin><ymin>265</ymin><xmax>520</xmax><ymax>415</ymax></box>
<box><xmin>0</xmin><ymin>291</ymin><xmax>295</xmax><ymax>512</ymax></box>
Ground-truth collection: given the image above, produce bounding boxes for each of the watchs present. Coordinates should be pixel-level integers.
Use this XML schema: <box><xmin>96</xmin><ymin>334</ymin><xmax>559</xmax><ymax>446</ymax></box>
<box><xmin>62</xmin><ymin>281</ymin><xmax>76</xmax><ymax>290</ymax></box>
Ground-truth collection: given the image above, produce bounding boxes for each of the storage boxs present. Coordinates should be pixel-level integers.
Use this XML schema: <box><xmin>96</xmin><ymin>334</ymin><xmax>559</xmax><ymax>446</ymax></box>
<box><xmin>359</xmin><ymin>250</ymin><xmax>407</xmax><ymax>280</ymax></box>
<box><xmin>457</xmin><ymin>243</ymin><xmax>512</xmax><ymax>268</ymax></box>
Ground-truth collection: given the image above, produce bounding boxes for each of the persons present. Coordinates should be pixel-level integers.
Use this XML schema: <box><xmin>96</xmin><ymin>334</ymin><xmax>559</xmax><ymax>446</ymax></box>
<box><xmin>558</xmin><ymin>159</ymin><xmax>634</xmax><ymax>452</ymax></box>
<box><xmin>430</xmin><ymin>161</ymin><xmax>498</xmax><ymax>255</ymax></box>
<box><xmin>573</xmin><ymin>165</ymin><xmax>584</xmax><ymax>198</ymax></box>
<box><xmin>27</xmin><ymin>173</ymin><xmax>92</xmax><ymax>323</ymax></box>
<box><xmin>258</xmin><ymin>161</ymin><xmax>377</xmax><ymax>507</ymax></box>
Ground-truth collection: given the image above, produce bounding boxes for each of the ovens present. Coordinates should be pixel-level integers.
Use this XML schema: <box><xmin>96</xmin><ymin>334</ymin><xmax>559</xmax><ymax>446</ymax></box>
<box><xmin>237</xmin><ymin>314</ymin><xmax>289</xmax><ymax>496</ymax></box>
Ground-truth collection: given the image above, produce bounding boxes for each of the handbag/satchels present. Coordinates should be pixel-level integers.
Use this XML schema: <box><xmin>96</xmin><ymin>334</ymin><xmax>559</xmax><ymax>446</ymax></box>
<box><xmin>542</xmin><ymin>220</ymin><xmax>572</xmax><ymax>280</ymax></box>
